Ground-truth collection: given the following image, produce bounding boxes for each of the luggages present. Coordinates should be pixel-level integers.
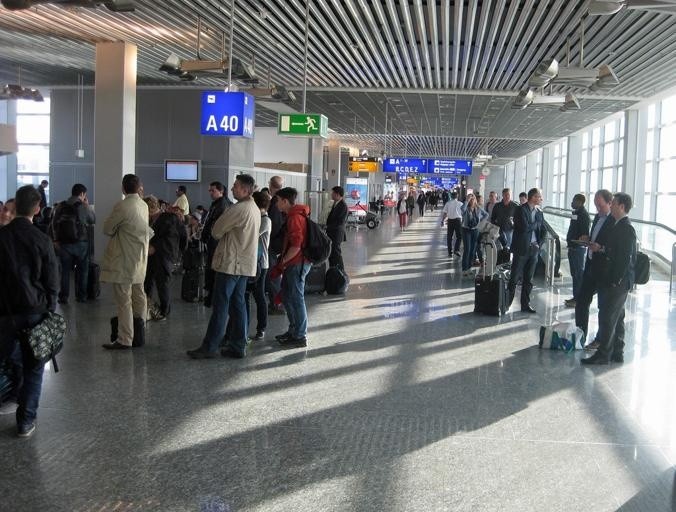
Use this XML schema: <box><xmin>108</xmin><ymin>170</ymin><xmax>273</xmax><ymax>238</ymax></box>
<box><xmin>475</xmin><ymin>243</ymin><xmax>506</xmax><ymax>315</ymax></box>
<box><xmin>74</xmin><ymin>240</ymin><xmax>101</xmax><ymax>302</ymax></box>
<box><xmin>181</xmin><ymin>238</ymin><xmax>202</xmax><ymax>303</ymax></box>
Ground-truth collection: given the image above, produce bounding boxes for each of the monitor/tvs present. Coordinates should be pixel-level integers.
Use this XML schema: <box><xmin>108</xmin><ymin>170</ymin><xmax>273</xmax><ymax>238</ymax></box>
<box><xmin>163</xmin><ymin>159</ymin><xmax>202</xmax><ymax>183</ymax></box>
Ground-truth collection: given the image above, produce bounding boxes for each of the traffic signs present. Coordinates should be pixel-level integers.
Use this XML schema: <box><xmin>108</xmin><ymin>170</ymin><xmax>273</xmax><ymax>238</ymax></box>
<box><xmin>427</xmin><ymin>159</ymin><xmax>473</xmax><ymax>175</ymax></box>
<box><xmin>279</xmin><ymin>114</ymin><xmax>321</xmax><ymax>135</ymax></box>
<box><xmin>384</xmin><ymin>156</ymin><xmax>429</xmax><ymax>175</ymax></box>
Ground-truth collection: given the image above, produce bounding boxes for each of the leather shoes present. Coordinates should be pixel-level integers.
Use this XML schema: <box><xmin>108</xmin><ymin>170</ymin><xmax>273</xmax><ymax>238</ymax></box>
<box><xmin>581</xmin><ymin>353</ymin><xmax>608</xmax><ymax>366</ymax></box>
<box><xmin>521</xmin><ymin>304</ymin><xmax>535</xmax><ymax>313</ymax></box>
<box><xmin>103</xmin><ymin>341</ymin><xmax>128</xmax><ymax>350</ymax></box>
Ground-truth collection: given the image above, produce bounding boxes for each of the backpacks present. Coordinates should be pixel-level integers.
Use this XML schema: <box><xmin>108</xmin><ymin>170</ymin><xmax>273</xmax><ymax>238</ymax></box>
<box><xmin>304</xmin><ymin>215</ymin><xmax>332</xmax><ymax>264</ymax></box>
<box><xmin>52</xmin><ymin>200</ymin><xmax>83</xmax><ymax>242</ymax></box>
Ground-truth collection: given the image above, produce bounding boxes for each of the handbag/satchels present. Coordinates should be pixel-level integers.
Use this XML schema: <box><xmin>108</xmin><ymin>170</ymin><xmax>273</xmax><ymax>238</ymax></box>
<box><xmin>111</xmin><ymin>315</ymin><xmax>145</xmax><ymax>346</ymax></box>
<box><xmin>635</xmin><ymin>252</ymin><xmax>650</xmax><ymax>284</ymax></box>
<box><xmin>327</xmin><ymin>265</ymin><xmax>349</xmax><ymax>295</ymax></box>
<box><xmin>26</xmin><ymin>311</ymin><xmax>67</xmax><ymax>371</ymax></box>
<box><xmin>540</xmin><ymin>321</ymin><xmax>585</xmax><ymax>352</ymax></box>
<box><xmin>183</xmin><ymin>243</ymin><xmax>202</xmax><ymax>272</ymax></box>
<box><xmin>306</xmin><ymin>267</ymin><xmax>326</xmax><ymax>296</ymax></box>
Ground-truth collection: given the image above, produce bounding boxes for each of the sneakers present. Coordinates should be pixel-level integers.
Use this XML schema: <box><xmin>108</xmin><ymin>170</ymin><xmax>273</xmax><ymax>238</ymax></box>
<box><xmin>586</xmin><ymin>340</ymin><xmax>600</xmax><ymax>349</ymax></box>
<box><xmin>274</xmin><ymin>332</ymin><xmax>291</xmax><ymax>341</ymax></box>
<box><xmin>282</xmin><ymin>338</ymin><xmax>306</xmax><ymax>347</ymax></box>
<box><xmin>17</xmin><ymin>421</ymin><xmax>36</xmax><ymax>436</ymax></box>
<box><xmin>221</xmin><ymin>347</ymin><xmax>243</xmax><ymax>357</ymax></box>
<box><xmin>256</xmin><ymin>332</ymin><xmax>265</xmax><ymax>340</ymax></box>
<box><xmin>187</xmin><ymin>349</ymin><xmax>217</xmax><ymax>359</ymax></box>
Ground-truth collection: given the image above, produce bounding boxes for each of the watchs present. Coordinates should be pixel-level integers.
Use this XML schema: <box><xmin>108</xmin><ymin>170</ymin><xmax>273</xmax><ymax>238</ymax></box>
<box><xmin>598</xmin><ymin>244</ymin><xmax>605</xmax><ymax>254</ymax></box>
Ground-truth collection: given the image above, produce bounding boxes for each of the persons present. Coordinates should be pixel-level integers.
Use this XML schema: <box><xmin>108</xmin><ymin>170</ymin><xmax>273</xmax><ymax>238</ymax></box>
<box><xmin>0</xmin><ymin>184</ymin><xmax>62</xmax><ymax>436</ymax></box>
<box><xmin>187</xmin><ymin>175</ymin><xmax>261</xmax><ymax>360</ymax></box>
<box><xmin>103</xmin><ymin>174</ymin><xmax>149</xmax><ymax>350</ymax></box>
<box><xmin>271</xmin><ymin>188</ymin><xmax>312</xmax><ymax>348</ymax></box>
<box><xmin>369</xmin><ymin>184</ymin><xmax>526</xmax><ymax>276</ymax></box>
<box><xmin>325</xmin><ymin>186</ymin><xmax>348</xmax><ymax>272</ymax></box>
<box><xmin>580</xmin><ymin>192</ymin><xmax>637</xmax><ymax>365</ymax></box>
<box><xmin>172</xmin><ymin>175</ymin><xmax>284</xmax><ymax>339</ymax></box>
<box><xmin>565</xmin><ymin>192</ymin><xmax>590</xmax><ymax>307</ymax></box>
<box><xmin>576</xmin><ymin>190</ymin><xmax>617</xmax><ymax>350</ymax></box>
<box><xmin>508</xmin><ymin>187</ymin><xmax>548</xmax><ymax>313</ymax></box>
<box><xmin>3</xmin><ymin>179</ymin><xmax>96</xmax><ymax>304</ymax></box>
<box><xmin>140</xmin><ymin>194</ymin><xmax>173</xmax><ymax>319</ymax></box>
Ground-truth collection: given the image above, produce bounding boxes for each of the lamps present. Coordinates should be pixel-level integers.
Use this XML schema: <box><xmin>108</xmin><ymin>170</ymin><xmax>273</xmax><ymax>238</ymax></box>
<box><xmin>507</xmin><ymin>0</ymin><xmax>676</xmax><ymax>111</ymax></box>
<box><xmin>472</xmin><ymin>120</ymin><xmax>492</xmax><ymax>169</ymax></box>
<box><xmin>0</xmin><ymin>58</ymin><xmax>46</xmax><ymax>101</ymax></box>
<box><xmin>157</xmin><ymin>13</ymin><xmax>297</xmax><ymax>103</ymax></box>
